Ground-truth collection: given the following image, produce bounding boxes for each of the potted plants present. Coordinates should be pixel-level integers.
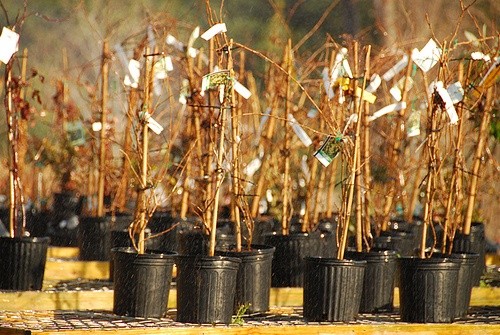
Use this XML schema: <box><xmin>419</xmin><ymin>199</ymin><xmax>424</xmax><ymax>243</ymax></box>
<box><xmin>1</xmin><ymin>0</ymin><xmax>500</xmax><ymax>326</ymax></box>
